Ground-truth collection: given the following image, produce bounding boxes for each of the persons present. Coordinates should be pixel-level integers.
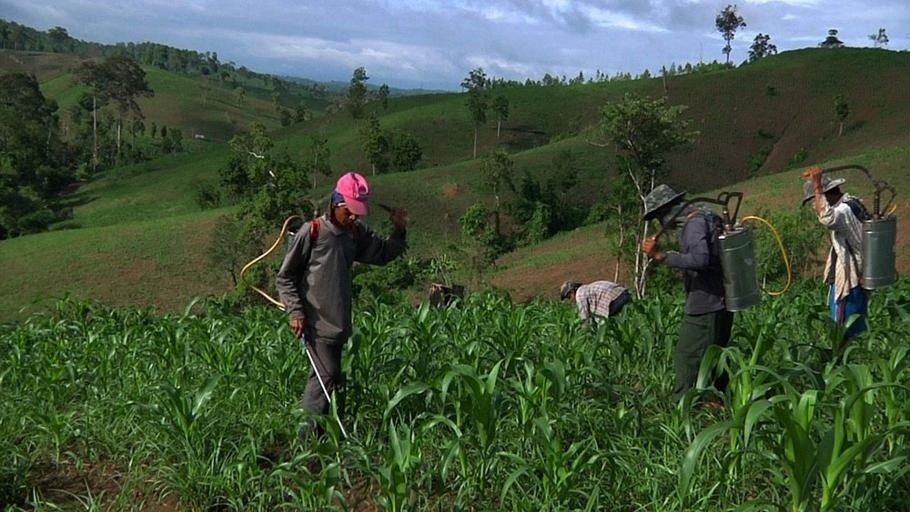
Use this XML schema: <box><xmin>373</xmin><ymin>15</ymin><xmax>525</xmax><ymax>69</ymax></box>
<box><xmin>642</xmin><ymin>184</ymin><xmax>733</xmax><ymax>399</ymax></box>
<box><xmin>277</xmin><ymin>173</ymin><xmax>409</xmax><ymax>423</ymax></box>
<box><xmin>799</xmin><ymin>166</ymin><xmax>873</xmax><ymax>349</ymax></box>
<box><xmin>560</xmin><ymin>280</ymin><xmax>633</xmax><ymax>327</ymax></box>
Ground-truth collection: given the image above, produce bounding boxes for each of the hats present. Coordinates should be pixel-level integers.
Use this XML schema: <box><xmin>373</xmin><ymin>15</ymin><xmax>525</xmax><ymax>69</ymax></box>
<box><xmin>642</xmin><ymin>184</ymin><xmax>687</xmax><ymax>220</ymax></box>
<box><xmin>561</xmin><ymin>281</ymin><xmax>577</xmax><ymax>300</ymax></box>
<box><xmin>335</xmin><ymin>171</ymin><xmax>370</xmax><ymax>216</ymax></box>
<box><xmin>803</xmin><ymin>174</ymin><xmax>846</xmax><ymax>207</ymax></box>
